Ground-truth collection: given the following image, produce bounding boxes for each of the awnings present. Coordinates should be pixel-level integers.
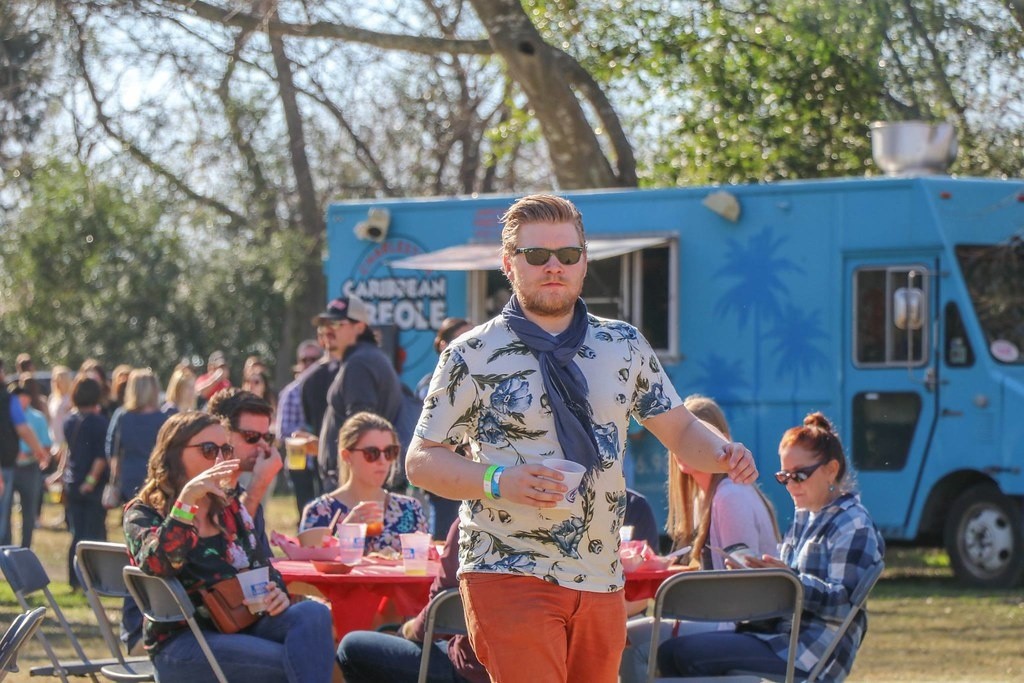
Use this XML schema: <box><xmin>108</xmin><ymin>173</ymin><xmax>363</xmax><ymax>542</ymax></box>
<box><xmin>391</xmin><ymin>236</ymin><xmax>677</xmax><ymax>270</ymax></box>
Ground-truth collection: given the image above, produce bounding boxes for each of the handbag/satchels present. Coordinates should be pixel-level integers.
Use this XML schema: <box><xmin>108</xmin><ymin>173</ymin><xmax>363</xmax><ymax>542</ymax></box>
<box><xmin>197</xmin><ymin>568</ymin><xmax>267</xmax><ymax>635</ymax></box>
<box><xmin>101</xmin><ymin>482</ymin><xmax>121</xmax><ymax>510</ymax></box>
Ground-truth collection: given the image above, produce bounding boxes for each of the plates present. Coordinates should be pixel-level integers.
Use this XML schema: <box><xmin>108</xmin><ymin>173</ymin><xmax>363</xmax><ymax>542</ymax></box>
<box><xmin>637</xmin><ymin>555</ymin><xmax>675</xmax><ymax>572</ymax></box>
<box><xmin>309</xmin><ymin>559</ymin><xmax>354</xmax><ymax>574</ymax></box>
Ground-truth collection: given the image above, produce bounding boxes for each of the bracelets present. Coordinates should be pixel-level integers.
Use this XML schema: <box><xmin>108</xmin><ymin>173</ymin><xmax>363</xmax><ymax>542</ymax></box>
<box><xmin>174</xmin><ymin>502</ymin><xmax>198</xmax><ymax>514</ymax></box>
<box><xmin>491</xmin><ymin>466</ymin><xmax>507</xmax><ymax>499</ymax></box>
<box><xmin>170</xmin><ymin>507</ymin><xmax>195</xmax><ymax>521</ymax></box>
<box><xmin>483</xmin><ymin>464</ymin><xmax>499</xmax><ymax>501</ymax></box>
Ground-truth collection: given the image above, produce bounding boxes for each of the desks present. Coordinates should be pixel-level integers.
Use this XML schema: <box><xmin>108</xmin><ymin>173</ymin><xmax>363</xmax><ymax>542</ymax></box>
<box><xmin>268</xmin><ymin>556</ymin><xmax>678</xmax><ymax>655</ymax></box>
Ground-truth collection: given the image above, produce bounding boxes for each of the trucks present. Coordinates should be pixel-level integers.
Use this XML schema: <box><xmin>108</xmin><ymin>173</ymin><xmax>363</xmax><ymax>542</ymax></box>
<box><xmin>325</xmin><ymin>119</ymin><xmax>1024</xmax><ymax>591</ymax></box>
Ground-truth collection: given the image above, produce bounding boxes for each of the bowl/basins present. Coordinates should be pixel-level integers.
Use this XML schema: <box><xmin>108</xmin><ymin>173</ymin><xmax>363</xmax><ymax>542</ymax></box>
<box><xmin>869</xmin><ymin>121</ymin><xmax>960</xmax><ymax>178</ymax></box>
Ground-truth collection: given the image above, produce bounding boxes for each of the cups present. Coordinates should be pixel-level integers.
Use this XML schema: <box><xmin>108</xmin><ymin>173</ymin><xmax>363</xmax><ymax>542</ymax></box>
<box><xmin>235</xmin><ymin>566</ymin><xmax>269</xmax><ymax>615</ymax></box>
<box><xmin>542</xmin><ymin>458</ymin><xmax>587</xmax><ymax>510</ymax></box>
<box><xmin>359</xmin><ymin>501</ymin><xmax>384</xmax><ymax>536</ymax></box>
<box><xmin>618</xmin><ymin>524</ymin><xmax>634</xmax><ymax>545</ymax></box>
<box><xmin>285</xmin><ymin>436</ymin><xmax>308</xmax><ymax>470</ymax></box>
<box><xmin>398</xmin><ymin>533</ymin><xmax>433</xmax><ymax>575</ymax></box>
<box><xmin>49</xmin><ymin>483</ymin><xmax>63</xmax><ymax>503</ymax></box>
<box><xmin>336</xmin><ymin>522</ymin><xmax>367</xmax><ymax>566</ymax></box>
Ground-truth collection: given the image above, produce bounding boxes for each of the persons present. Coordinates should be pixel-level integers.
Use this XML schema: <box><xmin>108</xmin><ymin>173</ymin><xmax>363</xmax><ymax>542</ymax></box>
<box><xmin>0</xmin><ymin>295</ymin><xmax>493</xmax><ymax>683</ymax></box>
<box><xmin>403</xmin><ymin>193</ymin><xmax>759</xmax><ymax>683</ymax></box>
<box><xmin>618</xmin><ymin>394</ymin><xmax>784</xmax><ymax>683</ymax></box>
<box><xmin>655</xmin><ymin>410</ymin><xmax>886</xmax><ymax>683</ymax></box>
<box><xmin>621</xmin><ymin>487</ymin><xmax>656</xmax><ymax>619</ymax></box>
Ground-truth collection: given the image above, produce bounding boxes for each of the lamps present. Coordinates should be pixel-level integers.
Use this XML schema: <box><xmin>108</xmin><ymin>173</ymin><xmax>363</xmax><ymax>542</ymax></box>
<box><xmin>702</xmin><ymin>189</ymin><xmax>743</xmax><ymax>223</ymax></box>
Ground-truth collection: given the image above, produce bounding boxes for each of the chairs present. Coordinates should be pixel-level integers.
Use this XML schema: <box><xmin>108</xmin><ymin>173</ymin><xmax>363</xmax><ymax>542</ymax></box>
<box><xmin>0</xmin><ymin>541</ymin><xmax>886</xmax><ymax>683</ymax></box>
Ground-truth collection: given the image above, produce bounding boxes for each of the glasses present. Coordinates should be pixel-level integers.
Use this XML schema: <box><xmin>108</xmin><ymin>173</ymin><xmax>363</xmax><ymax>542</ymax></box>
<box><xmin>184</xmin><ymin>441</ymin><xmax>234</xmax><ymax>462</ymax></box>
<box><xmin>774</xmin><ymin>456</ymin><xmax>832</xmax><ymax>485</ymax></box>
<box><xmin>298</xmin><ymin>357</ymin><xmax>319</xmax><ymax>364</ymax></box>
<box><xmin>512</xmin><ymin>241</ymin><xmax>584</xmax><ymax>266</ymax></box>
<box><xmin>347</xmin><ymin>443</ymin><xmax>400</xmax><ymax>463</ymax></box>
<box><xmin>317</xmin><ymin>319</ymin><xmax>359</xmax><ymax>330</ymax></box>
<box><xmin>228</xmin><ymin>426</ymin><xmax>276</xmax><ymax>446</ymax></box>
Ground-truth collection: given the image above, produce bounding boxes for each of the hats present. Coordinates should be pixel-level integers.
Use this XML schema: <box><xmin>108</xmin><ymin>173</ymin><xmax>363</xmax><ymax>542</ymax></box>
<box><xmin>319</xmin><ymin>296</ymin><xmax>377</xmax><ymax>326</ymax></box>
